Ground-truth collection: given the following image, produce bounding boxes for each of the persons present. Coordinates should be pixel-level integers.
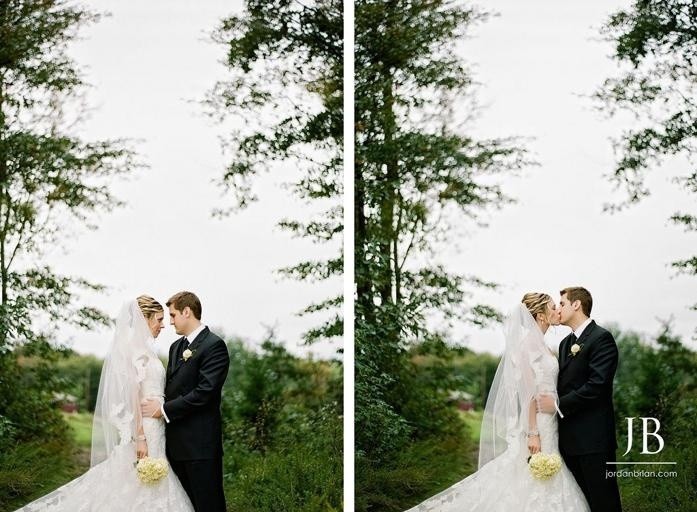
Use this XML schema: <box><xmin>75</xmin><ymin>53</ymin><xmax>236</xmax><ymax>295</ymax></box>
<box><xmin>475</xmin><ymin>291</ymin><xmax>593</xmax><ymax>512</ymax></box>
<box><xmin>140</xmin><ymin>290</ymin><xmax>231</xmax><ymax>512</ymax></box>
<box><xmin>531</xmin><ymin>286</ymin><xmax>624</xmax><ymax>512</ymax></box>
<box><xmin>90</xmin><ymin>293</ymin><xmax>199</xmax><ymax>512</ymax></box>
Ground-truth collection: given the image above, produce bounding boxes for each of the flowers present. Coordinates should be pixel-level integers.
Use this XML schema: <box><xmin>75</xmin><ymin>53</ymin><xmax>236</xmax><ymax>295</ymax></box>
<box><xmin>179</xmin><ymin>347</ymin><xmax>197</xmax><ymax>363</ymax></box>
<box><xmin>566</xmin><ymin>343</ymin><xmax>583</xmax><ymax>358</ymax></box>
<box><xmin>136</xmin><ymin>456</ymin><xmax>169</xmax><ymax>485</ymax></box>
<box><xmin>526</xmin><ymin>451</ymin><xmax>562</xmax><ymax>481</ymax></box>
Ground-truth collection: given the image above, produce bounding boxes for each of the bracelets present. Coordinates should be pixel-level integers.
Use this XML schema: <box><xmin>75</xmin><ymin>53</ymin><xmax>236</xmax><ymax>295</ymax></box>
<box><xmin>134</xmin><ymin>435</ymin><xmax>146</xmax><ymax>443</ymax></box>
<box><xmin>527</xmin><ymin>431</ymin><xmax>540</xmax><ymax>439</ymax></box>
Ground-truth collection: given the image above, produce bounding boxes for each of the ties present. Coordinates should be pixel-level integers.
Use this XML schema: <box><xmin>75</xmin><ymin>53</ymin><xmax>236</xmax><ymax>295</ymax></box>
<box><xmin>571</xmin><ymin>333</ymin><xmax>577</xmax><ymax>345</ymax></box>
<box><xmin>179</xmin><ymin>338</ymin><xmax>189</xmax><ymax>358</ymax></box>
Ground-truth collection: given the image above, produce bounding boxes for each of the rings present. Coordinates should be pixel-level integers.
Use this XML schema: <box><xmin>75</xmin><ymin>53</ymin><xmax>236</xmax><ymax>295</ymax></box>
<box><xmin>529</xmin><ymin>447</ymin><xmax>530</xmax><ymax>449</ymax></box>
<box><xmin>136</xmin><ymin>456</ymin><xmax>139</xmax><ymax>457</ymax></box>
